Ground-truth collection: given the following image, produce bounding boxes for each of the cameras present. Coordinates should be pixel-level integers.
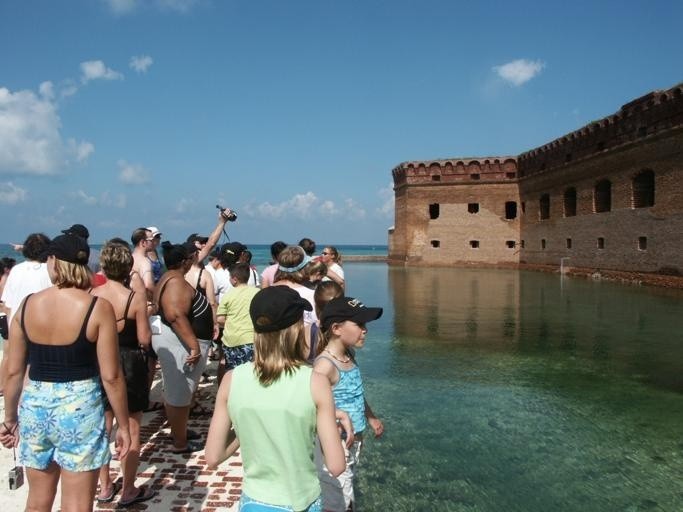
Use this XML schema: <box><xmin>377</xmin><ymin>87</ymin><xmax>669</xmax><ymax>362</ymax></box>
<box><xmin>217</xmin><ymin>204</ymin><xmax>238</xmax><ymax>225</ymax></box>
<box><xmin>8</xmin><ymin>464</ymin><xmax>25</xmax><ymax>490</ymax></box>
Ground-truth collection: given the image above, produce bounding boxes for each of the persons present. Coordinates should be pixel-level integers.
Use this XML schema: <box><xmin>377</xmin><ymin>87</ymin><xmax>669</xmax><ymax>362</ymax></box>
<box><xmin>314</xmin><ymin>296</ymin><xmax>383</xmax><ymax>510</ymax></box>
<box><xmin>0</xmin><ymin>222</ymin><xmax>163</xmax><ymax>411</ymax></box>
<box><xmin>204</xmin><ymin>285</ymin><xmax>346</xmax><ymax>512</ymax></box>
<box><xmin>150</xmin><ymin>241</ymin><xmax>212</xmax><ymax>455</ymax></box>
<box><xmin>0</xmin><ymin>234</ymin><xmax>132</xmax><ymax>512</ymax></box>
<box><xmin>183</xmin><ymin>208</ymin><xmax>344</xmax><ymax>390</ymax></box>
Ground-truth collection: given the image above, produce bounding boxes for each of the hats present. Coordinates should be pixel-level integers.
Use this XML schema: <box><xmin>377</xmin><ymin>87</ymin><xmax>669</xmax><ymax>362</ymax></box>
<box><xmin>249</xmin><ymin>286</ymin><xmax>312</xmax><ymax>330</ymax></box>
<box><xmin>38</xmin><ymin>223</ymin><xmax>312</xmax><ymax>272</ymax></box>
<box><xmin>320</xmin><ymin>297</ymin><xmax>383</xmax><ymax>332</ymax></box>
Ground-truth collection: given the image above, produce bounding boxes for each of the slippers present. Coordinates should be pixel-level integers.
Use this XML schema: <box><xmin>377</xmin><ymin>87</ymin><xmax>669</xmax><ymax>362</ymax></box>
<box><xmin>112</xmin><ymin>352</ymin><xmax>219</xmax><ymax>454</ymax></box>
<box><xmin>118</xmin><ymin>487</ymin><xmax>155</xmax><ymax>506</ymax></box>
<box><xmin>98</xmin><ymin>483</ymin><xmax>121</xmax><ymax>502</ymax></box>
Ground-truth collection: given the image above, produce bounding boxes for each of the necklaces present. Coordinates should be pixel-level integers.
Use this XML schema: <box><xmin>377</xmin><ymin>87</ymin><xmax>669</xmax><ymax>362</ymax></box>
<box><xmin>325</xmin><ymin>345</ymin><xmax>351</xmax><ymax>363</ymax></box>
